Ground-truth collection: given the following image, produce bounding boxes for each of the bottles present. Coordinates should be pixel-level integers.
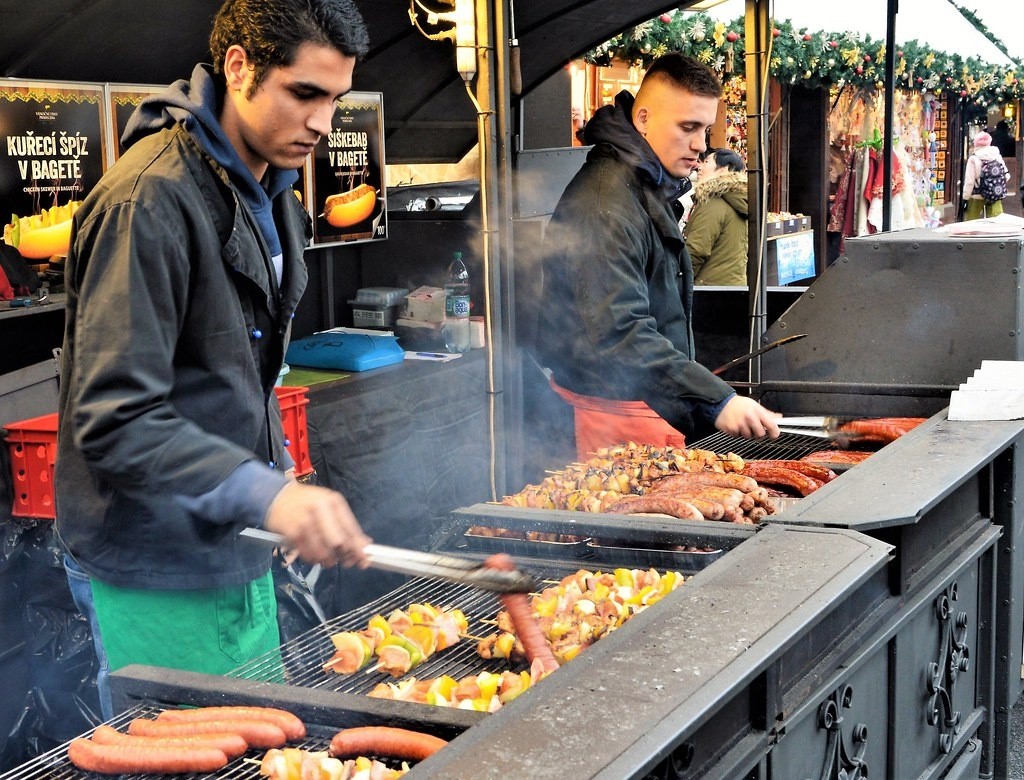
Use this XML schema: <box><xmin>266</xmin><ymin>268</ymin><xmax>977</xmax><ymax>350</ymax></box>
<box><xmin>442</xmin><ymin>251</ymin><xmax>471</xmax><ymax>354</ymax></box>
<box><xmin>357</xmin><ymin>287</ymin><xmax>409</xmax><ymax>306</ymax></box>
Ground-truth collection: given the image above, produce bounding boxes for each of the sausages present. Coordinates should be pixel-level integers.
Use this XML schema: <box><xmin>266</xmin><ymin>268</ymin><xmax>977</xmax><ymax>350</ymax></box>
<box><xmin>840</xmin><ymin>418</ymin><xmax>928</xmax><ymax>441</ymax></box>
<box><xmin>68</xmin><ymin>706</ymin><xmax>306</xmax><ymax>774</ymax></box>
<box><xmin>590</xmin><ymin>460</ymin><xmax>838</xmax><ymax>552</ymax></box>
<box><xmin>326</xmin><ymin>726</ymin><xmax>450</xmax><ymax>760</ymax></box>
<box><xmin>486</xmin><ymin>553</ymin><xmax>559</xmax><ymax>674</ymax></box>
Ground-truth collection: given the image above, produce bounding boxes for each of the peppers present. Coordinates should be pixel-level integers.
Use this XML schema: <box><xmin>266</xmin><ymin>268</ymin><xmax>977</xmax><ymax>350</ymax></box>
<box><xmin>342</xmin><ymin>566</ymin><xmax>677</xmax><ymax>711</ymax></box>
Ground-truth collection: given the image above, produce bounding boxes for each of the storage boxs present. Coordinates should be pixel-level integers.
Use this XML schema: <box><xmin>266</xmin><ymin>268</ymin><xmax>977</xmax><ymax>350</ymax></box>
<box><xmin>767</xmin><ymin>220</ymin><xmax>784</xmax><ymax>236</ymax></box>
<box><xmin>1</xmin><ymin>386</ymin><xmax>314</xmax><ymax>519</ymax></box>
<box><xmin>783</xmin><ymin>219</ymin><xmax>798</xmax><ymax>234</ymax></box>
<box><xmin>797</xmin><ymin>217</ymin><xmax>812</xmax><ymax>231</ymax></box>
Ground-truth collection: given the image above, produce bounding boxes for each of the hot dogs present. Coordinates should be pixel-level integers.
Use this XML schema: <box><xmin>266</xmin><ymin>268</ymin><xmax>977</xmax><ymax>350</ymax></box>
<box><xmin>323</xmin><ymin>183</ymin><xmax>376</xmax><ymax>227</ymax></box>
<box><xmin>2</xmin><ymin>200</ymin><xmax>83</xmax><ymax>258</ymax></box>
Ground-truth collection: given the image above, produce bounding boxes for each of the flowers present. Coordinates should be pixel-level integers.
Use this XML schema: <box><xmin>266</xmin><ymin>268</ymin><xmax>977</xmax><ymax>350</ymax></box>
<box><xmin>578</xmin><ymin>9</ymin><xmax>1024</xmax><ymax>107</ymax></box>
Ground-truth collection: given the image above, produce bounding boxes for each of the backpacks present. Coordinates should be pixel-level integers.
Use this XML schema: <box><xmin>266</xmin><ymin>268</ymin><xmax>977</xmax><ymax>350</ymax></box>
<box><xmin>972</xmin><ymin>153</ymin><xmax>1008</xmax><ymax>202</ymax></box>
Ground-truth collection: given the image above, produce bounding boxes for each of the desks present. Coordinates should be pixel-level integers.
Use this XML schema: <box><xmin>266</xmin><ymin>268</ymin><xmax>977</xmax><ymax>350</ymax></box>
<box><xmin>766</xmin><ymin>229</ymin><xmax>814</xmax><ymax>284</ymax></box>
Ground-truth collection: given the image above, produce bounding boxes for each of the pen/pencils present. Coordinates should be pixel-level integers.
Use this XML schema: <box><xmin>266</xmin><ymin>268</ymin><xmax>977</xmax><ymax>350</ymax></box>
<box><xmin>416</xmin><ymin>352</ymin><xmax>447</xmax><ymax>358</ymax></box>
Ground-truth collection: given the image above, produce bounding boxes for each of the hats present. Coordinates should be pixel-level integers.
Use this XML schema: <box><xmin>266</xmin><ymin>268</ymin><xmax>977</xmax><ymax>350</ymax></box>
<box><xmin>974</xmin><ymin>131</ymin><xmax>992</xmax><ymax>147</ymax></box>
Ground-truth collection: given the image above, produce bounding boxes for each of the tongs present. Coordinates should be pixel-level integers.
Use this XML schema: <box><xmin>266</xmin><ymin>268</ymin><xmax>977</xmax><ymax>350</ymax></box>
<box><xmin>362</xmin><ymin>541</ymin><xmax>543</xmax><ymax>593</ymax></box>
<box><xmin>763</xmin><ymin>415</ymin><xmax>881</xmax><ymax>439</ymax></box>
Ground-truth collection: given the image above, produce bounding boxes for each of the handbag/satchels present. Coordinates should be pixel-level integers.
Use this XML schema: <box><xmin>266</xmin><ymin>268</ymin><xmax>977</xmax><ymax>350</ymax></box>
<box><xmin>285</xmin><ymin>331</ymin><xmax>405</xmax><ymax>372</ymax></box>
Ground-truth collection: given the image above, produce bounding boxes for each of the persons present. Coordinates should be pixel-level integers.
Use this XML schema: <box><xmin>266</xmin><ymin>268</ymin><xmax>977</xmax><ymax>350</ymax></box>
<box><xmin>680</xmin><ymin>147</ymin><xmax>747</xmax><ymax>287</ymax></box>
<box><xmin>962</xmin><ymin>131</ymin><xmax>1011</xmax><ymax>220</ymax></box>
<box><xmin>535</xmin><ymin>52</ymin><xmax>782</xmax><ymax>483</ymax></box>
<box><xmin>54</xmin><ymin>1</ymin><xmax>373</xmax><ymax>722</ymax></box>
